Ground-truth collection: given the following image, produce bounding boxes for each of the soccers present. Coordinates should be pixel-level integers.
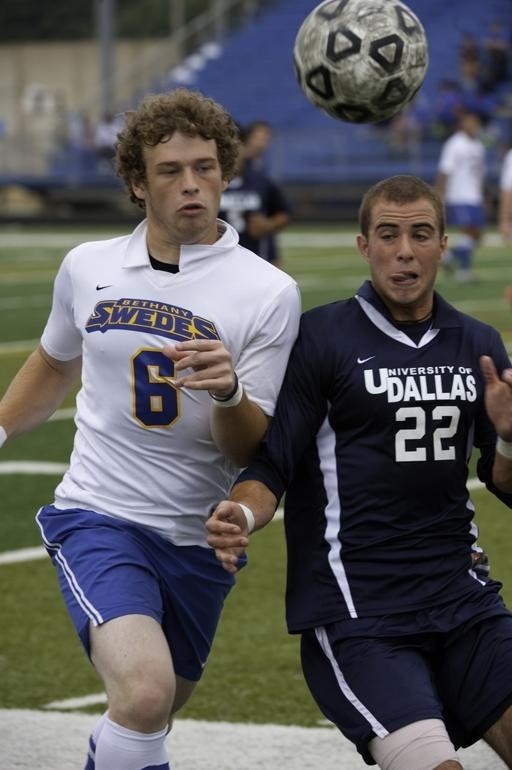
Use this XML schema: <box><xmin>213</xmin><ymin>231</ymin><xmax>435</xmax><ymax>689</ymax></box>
<box><xmin>292</xmin><ymin>0</ymin><xmax>428</xmax><ymax>123</ymax></box>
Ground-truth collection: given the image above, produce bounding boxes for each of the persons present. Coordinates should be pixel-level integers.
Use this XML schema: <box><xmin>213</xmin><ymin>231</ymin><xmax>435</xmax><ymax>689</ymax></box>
<box><xmin>205</xmin><ymin>170</ymin><xmax>512</xmax><ymax>770</ymax></box>
<box><xmin>0</xmin><ymin>88</ymin><xmax>302</xmax><ymax>770</ymax></box>
<box><xmin>496</xmin><ymin>150</ymin><xmax>512</xmax><ymax>307</ymax></box>
<box><xmin>216</xmin><ymin>121</ymin><xmax>293</xmax><ymax>262</ymax></box>
<box><xmin>382</xmin><ymin>20</ymin><xmax>511</xmax><ymax>156</ymax></box>
<box><xmin>434</xmin><ymin>111</ymin><xmax>487</xmax><ymax>286</ymax></box>
<box><xmin>244</xmin><ymin>120</ymin><xmax>283</xmax><ymax>271</ymax></box>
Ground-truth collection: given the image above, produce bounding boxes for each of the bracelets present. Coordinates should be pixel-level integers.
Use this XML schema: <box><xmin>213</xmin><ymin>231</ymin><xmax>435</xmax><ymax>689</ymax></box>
<box><xmin>206</xmin><ymin>372</ymin><xmax>239</xmax><ymax>402</ymax></box>
<box><xmin>208</xmin><ymin>383</ymin><xmax>244</xmax><ymax>410</ymax></box>
<box><xmin>236</xmin><ymin>501</ymin><xmax>257</xmax><ymax>536</ymax></box>
<box><xmin>0</xmin><ymin>425</ymin><xmax>11</xmax><ymax>447</ymax></box>
<box><xmin>495</xmin><ymin>436</ymin><xmax>511</xmax><ymax>461</ymax></box>
